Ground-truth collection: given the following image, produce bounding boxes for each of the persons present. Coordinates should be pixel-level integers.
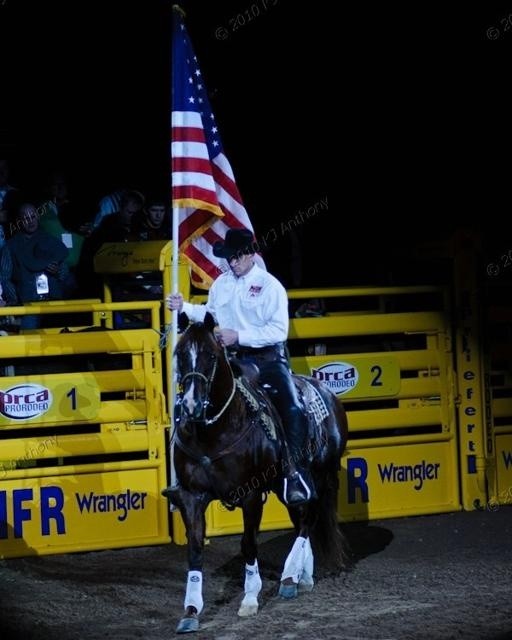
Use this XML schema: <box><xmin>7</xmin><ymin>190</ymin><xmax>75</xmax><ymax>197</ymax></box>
<box><xmin>159</xmin><ymin>226</ymin><xmax>319</xmax><ymax>505</ymax></box>
<box><xmin>1</xmin><ymin>154</ymin><xmax>172</xmax><ymax>467</ymax></box>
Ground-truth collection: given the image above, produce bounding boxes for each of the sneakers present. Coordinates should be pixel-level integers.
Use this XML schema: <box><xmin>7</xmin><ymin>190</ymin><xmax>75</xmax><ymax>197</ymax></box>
<box><xmin>287</xmin><ymin>478</ymin><xmax>307</xmax><ymax>502</ymax></box>
<box><xmin>162</xmin><ymin>484</ymin><xmax>182</xmax><ymax>505</ymax></box>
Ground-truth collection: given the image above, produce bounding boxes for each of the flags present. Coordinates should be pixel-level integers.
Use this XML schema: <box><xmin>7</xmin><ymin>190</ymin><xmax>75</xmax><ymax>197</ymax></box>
<box><xmin>170</xmin><ymin>6</ymin><xmax>266</xmax><ymax>280</ymax></box>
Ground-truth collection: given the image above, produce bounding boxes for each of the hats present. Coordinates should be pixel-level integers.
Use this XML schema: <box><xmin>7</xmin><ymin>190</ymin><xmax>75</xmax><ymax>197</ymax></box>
<box><xmin>213</xmin><ymin>227</ymin><xmax>260</xmax><ymax>258</ymax></box>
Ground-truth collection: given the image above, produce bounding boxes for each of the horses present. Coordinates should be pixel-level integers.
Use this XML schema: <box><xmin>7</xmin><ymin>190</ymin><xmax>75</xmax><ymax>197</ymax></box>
<box><xmin>173</xmin><ymin>311</ymin><xmax>349</xmax><ymax>634</ymax></box>
